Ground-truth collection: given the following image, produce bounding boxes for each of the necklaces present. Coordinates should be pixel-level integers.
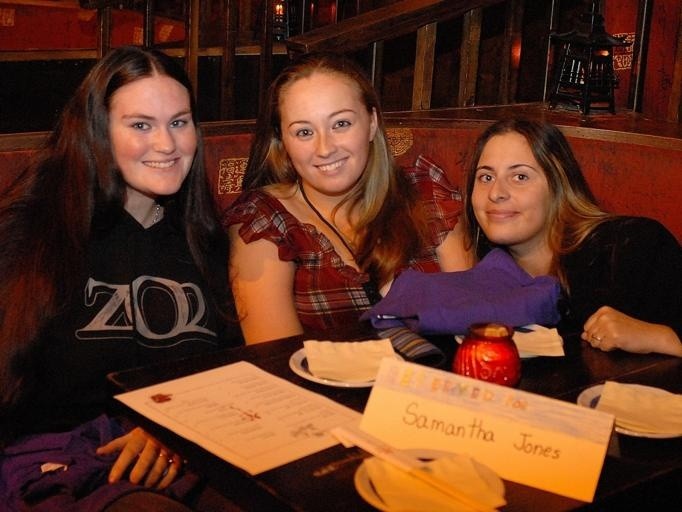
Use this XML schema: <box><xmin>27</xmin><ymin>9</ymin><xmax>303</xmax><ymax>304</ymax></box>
<box><xmin>146</xmin><ymin>200</ymin><xmax>164</xmax><ymax>226</ymax></box>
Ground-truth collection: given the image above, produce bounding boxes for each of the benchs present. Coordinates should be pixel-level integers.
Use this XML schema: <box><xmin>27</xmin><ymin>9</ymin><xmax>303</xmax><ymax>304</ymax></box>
<box><xmin>0</xmin><ymin>118</ymin><xmax>681</xmax><ymax>251</ymax></box>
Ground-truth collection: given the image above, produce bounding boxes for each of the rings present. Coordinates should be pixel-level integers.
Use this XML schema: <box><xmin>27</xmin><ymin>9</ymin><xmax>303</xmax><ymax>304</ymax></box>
<box><xmin>592</xmin><ymin>332</ymin><xmax>606</xmax><ymax>343</ymax></box>
<box><xmin>153</xmin><ymin>448</ymin><xmax>173</xmax><ymax>466</ymax></box>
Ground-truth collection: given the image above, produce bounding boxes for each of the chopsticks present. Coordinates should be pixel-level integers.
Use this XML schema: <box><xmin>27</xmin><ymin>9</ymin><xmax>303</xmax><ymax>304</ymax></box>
<box><xmin>329</xmin><ymin>426</ymin><xmax>500</xmax><ymax>512</ymax></box>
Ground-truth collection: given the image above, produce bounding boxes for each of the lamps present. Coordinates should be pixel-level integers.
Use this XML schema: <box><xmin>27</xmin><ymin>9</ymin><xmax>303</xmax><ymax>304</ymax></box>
<box><xmin>254</xmin><ymin>0</ymin><xmax>308</xmax><ymax>41</ymax></box>
<box><xmin>545</xmin><ymin>0</ymin><xmax>633</xmax><ymax>121</ymax></box>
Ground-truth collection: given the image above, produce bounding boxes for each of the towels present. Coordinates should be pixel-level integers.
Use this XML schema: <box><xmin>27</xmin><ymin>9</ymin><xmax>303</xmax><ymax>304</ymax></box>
<box><xmin>299</xmin><ymin>336</ymin><xmax>399</xmax><ymax>385</ymax></box>
<box><xmin>594</xmin><ymin>376</ymin><xmax>681</xmax><ymax>437</ymax></box>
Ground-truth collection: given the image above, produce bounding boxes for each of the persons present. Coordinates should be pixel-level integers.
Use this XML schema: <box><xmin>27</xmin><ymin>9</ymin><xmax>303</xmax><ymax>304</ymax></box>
<box><xmin>221</xmin><ymin>53</ymin><xmax>484</xmax><ymax>352</ymax></box>
<box><xmin>0</xmin><ymin>40</ymin><xmax>231</xmax><ymax>511</ymax></box>
<box><xmin>460</xmin><ymin>115</ymin><xmax>682</xmax><ymax>357</ymax></box>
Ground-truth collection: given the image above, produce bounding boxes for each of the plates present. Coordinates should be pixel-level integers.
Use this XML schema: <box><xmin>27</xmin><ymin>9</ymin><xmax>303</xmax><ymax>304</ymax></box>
<box><xmin>352</xmin><ymin>447</ymin><xmax>508</xmax><ymax>512</ymax></box>
<box><xmin>577</xmin><ymin>381</ymin><xmax>681</xmax><ymax>440</ymax></box>
<box><xmin>287</xmin><ymin>347</ymin><xmax>407</xmax><ymax>388</ymax></box>
<box><xmin>452</xmin><ymin>321</ymin><xmax>564</xmax><ymax>360</ymax></box>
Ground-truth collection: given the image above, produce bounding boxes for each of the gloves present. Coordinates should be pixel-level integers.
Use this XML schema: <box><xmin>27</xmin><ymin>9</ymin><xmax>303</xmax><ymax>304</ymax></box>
<box><xmin>6</xmin><ymin>414</ymin><xmax>124</xmax><ymax>500</ymax></box>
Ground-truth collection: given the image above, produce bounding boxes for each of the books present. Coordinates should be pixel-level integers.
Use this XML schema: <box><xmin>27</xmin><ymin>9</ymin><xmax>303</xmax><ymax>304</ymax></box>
<box><xmin>560</xmin><ymin>54</ymin><xmax>612</xmax><ymax>85</ymax></box>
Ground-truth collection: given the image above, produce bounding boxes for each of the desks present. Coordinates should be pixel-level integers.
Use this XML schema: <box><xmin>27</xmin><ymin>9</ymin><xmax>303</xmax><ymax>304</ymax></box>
<box><xmin>104</xmin><ymin>314</ymin><xmax>682</xmax><ymax>512</ymax></box>
